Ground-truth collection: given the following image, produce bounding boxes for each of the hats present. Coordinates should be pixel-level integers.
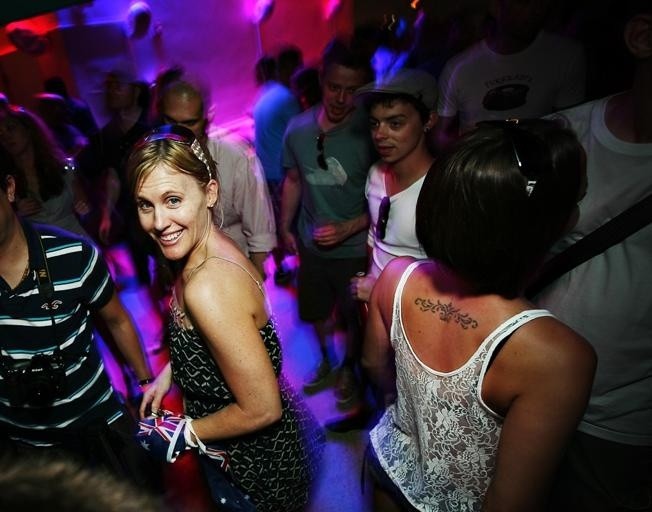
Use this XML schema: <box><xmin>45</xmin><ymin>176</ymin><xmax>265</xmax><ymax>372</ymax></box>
<box><xmin>355</xmin><ymin>69</ymin><xmax>438</xmax><ymax>108</ymax></box>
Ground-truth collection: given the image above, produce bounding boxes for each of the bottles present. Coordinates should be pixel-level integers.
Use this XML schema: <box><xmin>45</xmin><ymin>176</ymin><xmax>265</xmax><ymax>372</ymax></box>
<box><xmin>350</xmin><ymin>272</ymin><xmax>369</xmax><ymax>328</ymax></box>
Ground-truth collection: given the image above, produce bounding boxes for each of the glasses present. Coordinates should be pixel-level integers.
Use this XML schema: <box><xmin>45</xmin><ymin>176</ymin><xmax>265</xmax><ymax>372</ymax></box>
<box><xmin>477</xmin><ymin>118</ymin><xmax>552</xmax><ymax>197</ymax></box>
<box><xmin>376</xmin><ymin>197</ymin><xmax>389</xmax><ymax>238</ymax></box>
<box><xmin>317</xmin><ymin>134</ymin><xmax>328</xmax><ymax>171</ymax></box>
<box><xmin>133</xmin><ymin>124</ymin><xmax>212</xmax><ymax>181</ymax></box>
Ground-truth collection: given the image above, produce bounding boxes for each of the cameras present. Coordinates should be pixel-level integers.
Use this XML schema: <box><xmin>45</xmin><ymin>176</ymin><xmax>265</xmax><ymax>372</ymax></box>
<box><xmin>3</xmin><ymin>354</ymin><xmax>65</xmax><ymax>408</ymax></box>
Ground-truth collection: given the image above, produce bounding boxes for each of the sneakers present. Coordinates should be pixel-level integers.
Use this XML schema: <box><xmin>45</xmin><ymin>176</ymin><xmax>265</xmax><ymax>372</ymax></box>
<box><xmin>303</xmin><ymin>359</ymin><xmax>339</xmax><ymax>388</ymax></box>
<box><xmin>335</xmin><ymin>368</ymin><xmax>355</xmax><ymax>403</ymax></box>
<box><xmin>325</xmin><ymin>409</ymin><xmax>373</xmax><ymax>433</ymax></box>
